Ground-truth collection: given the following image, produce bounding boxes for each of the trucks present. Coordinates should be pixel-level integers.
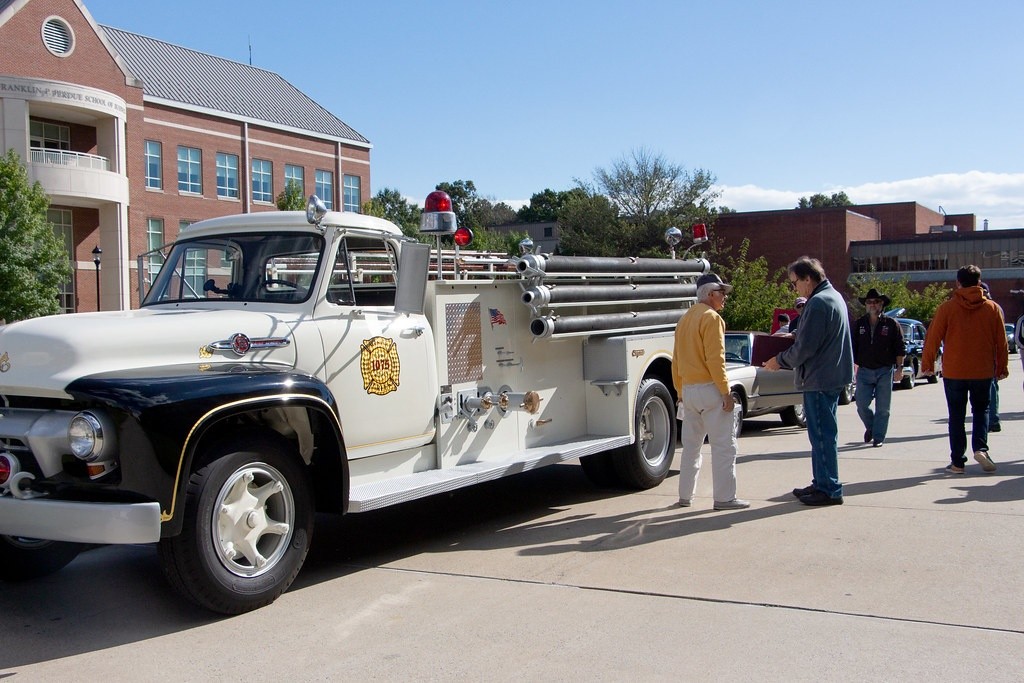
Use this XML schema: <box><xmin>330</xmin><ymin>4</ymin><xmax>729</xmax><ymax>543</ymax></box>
<box><xmin>0</xmin><ymin>185</ymin><xmax>713</xmax><ymax>616</ymax></box>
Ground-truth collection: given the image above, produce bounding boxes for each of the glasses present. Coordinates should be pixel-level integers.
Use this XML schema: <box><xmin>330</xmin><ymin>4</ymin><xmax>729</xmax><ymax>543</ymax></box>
<box><xmin>791</xmin><ymin>279</ymin><xmax>799</xmax><ymax>287</ymax></box>
<box><xmin>866</xmin><ymin>299</ymin><xmax>882</xmax><ymax>304</ymax></box>
<box><xmin>797</xmin><ymin>303</ymin><xmax>804</xmax><ymax>308</ymax></box>
<box><xmin>713</xmin><ymin>289</ymin><xmax>725</xmax><ymax>296</ymax></box>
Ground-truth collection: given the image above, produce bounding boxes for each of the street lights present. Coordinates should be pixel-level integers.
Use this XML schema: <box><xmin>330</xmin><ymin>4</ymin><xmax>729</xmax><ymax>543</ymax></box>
<box><xmin>91</xmin><ymin>244</ymin><xmax>102</xmax><ymax>311</ymax></box>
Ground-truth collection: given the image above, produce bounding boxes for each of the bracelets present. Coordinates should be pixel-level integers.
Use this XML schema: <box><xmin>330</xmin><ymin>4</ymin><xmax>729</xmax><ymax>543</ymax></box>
<box><xmin>898</xmin><ymin>364</ymin><xmax>904</xmax><ymax>368</ymax></box>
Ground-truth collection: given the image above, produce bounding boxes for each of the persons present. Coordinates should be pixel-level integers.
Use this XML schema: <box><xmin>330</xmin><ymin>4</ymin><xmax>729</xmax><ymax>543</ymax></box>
<box><xmin>1014</xmin><ymin>314</ymin><xmax>1024</xmax><ymax>370</ymax></box>
<box><xmin>922</xmin><ymin>266</ymin><xmax>1009</xmax><ymax>473</ymax></box>
<box><xmin>979</xmin><ymin>281</ymin><xmax>1006</xmax><ymax>432</ymax></box>
<box><xmin>775</xmin><ymin>313</ymin><xmax>791</xmax><ymax>333</ymax></box>
<box><xmin>851</xmin><ymin>289</ymin><xmax>908</xmax><ymax>447</ymax></box>
<box><xmin>762</xmin><ymin>256</ymin><xmax>854</xmax><ymax>506</ymax></box>
<box><xmin>671</xmin><ymin>273</ymin><xmax>750</xmax><ymax>510</ymax></box>
<box><xmin>789</xmin><ymin>297</ymin><xmax>807</xmax><ymax>333</ymax></box>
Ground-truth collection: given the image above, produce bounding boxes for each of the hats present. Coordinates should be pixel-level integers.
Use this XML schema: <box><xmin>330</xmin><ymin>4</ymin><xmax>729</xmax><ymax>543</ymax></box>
<box><xmin>980</xmin><ymin>282</ymin><xmax>989</xmax><ymax>293</ymax></box>
<box><xmin>795</xmin><ymin>297</ymin><xmax>807</xmax><ymax>307</ymax></box>
<box><xmin>697</xmin><ymin>274</ymin><xmax>732</xmax><ymax>294</ymax></box>
<box><xmin>858</xmin><ymin>289</ymin><xmax>890</xmax><ymax>306</ymax></box>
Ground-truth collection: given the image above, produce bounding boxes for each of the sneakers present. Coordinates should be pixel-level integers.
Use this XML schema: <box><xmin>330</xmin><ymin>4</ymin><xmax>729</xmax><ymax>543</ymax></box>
<box><xmin>974</xmin><ymin>450</ymin><xmax>998</xmax><ymax>473</ymax></box>
<box><xmin>792</xmin><ymin>484</ymin><xmax>818</xmax><ymax>498</ymax></box>
<box><xmin>800</xmin><ymin>489</ymin><xmax>844</xmax><ymax>506</ymax></box>
<box><xmin>946</xmin><ymin>465</ymin><xmax>964</xmax><ymax>474</ymax></box>
<box><xmin>714</xmin><ymin>496</ymin><xmax>750</xmax><ymax>509</ymax></box>
<box><xmin>679</xmin><ymin>498</ymin><xmax>692</xmax><ymax>507</ymax></box>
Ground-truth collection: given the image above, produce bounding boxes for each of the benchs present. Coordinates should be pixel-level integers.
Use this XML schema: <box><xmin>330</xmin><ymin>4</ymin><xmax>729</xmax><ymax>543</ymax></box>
<box><xmin>264</xmin><ymin>288</ymin><xmax>396</xmax><ymax>305</ymax></box>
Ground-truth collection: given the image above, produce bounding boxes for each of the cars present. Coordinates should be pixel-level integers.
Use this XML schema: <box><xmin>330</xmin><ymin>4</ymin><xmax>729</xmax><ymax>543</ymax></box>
<box><xmin>769</xmin><ymin>305</ymin><xmax>859</xmax><ymax>404</ymax></box>
<box><xmin>885</xmin><ymin>308</ymin><xmax>941</xmax><ymax>390</ymax></box>
<box><xmin>723</xmin><ymin>331</ymin><xmax>807</xmax><ymax>437</ymax></box>
<box><xmin>1005</xmin><ymin>324</ymin><xmax>1020</xmax><ymax>354</ymax></box>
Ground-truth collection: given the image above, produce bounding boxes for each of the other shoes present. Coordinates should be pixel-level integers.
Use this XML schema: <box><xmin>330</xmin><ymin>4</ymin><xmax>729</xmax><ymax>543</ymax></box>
<box><xmin>864</xmin><ymin>430</ymin><xmax>872</xmax><ymax>442</ymax></box>
<box><xmin>873</xmin><ymin>441</ymin><xmax>883</xmax><ymax>447</ymax></box>
<box><xmin>988</xmin><ymin>424</ymin><xmax>1001</xmax><ymax>432</ymax></box>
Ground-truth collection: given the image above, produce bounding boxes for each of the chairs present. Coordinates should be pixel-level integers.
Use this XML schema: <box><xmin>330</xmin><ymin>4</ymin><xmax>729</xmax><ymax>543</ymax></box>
<box><xmin>741</xmin><ymin>346</ymin><xmax>748</xmax><ymax>361</ymax></box>
